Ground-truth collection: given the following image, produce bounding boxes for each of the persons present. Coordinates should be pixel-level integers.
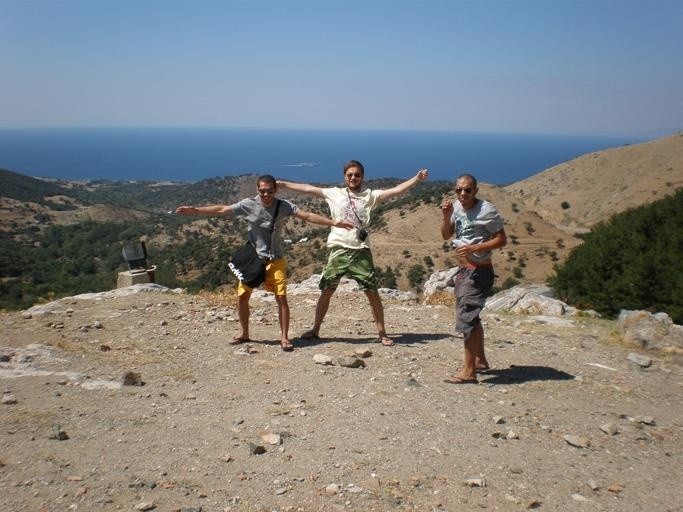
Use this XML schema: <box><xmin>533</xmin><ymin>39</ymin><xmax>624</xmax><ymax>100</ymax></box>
<box><xmin>175</xmin><ymin>173</ymin><xmax>353</xmax><ymax>351</ymax></box>
<box><xmin>273</xmin><ymin>160</ymin><xmax>429</xmax><ymax>346</ymax></box>
<box><xmin>440</xmin><ymin>174</ymin><xmax>507</xmax><ymax>384</ymax></box>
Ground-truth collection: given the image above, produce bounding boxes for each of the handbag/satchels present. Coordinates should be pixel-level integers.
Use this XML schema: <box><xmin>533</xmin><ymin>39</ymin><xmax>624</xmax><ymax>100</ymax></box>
<box><xmin>227</xmin><ymin>241</ymin><xmax>267</xmax><ymax>288</ymax></box>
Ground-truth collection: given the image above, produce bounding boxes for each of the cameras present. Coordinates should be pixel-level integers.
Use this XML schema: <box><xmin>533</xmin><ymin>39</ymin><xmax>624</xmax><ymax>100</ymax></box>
<box><xmin>359</xmin><ymin>229</ymin><xmax>367</xmax><ymax>241</ymax></box>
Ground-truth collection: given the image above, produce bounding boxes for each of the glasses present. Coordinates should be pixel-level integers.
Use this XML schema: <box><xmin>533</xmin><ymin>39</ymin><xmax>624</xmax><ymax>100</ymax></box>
<box><xmin>346</xmin><ymin>173</ymin><xmax>361</xmax><ymax>179</ymax></box>
<box><xmin>257</xmin><ymin>186</ymin><xmax>275</xmax><ymax>195</ymax></box>
<box><xmin>455</xmin><ymin>187</ymin><xmax>472</xmax><ymax>193</ymax></box>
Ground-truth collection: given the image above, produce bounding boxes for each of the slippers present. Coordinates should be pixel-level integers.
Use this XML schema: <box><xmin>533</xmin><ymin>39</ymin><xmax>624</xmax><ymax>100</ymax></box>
<box><xmin>280</xmin><ymin>338</ymin><xmax>294</xmax><ymax>349</ymax></box>
<box><xmin>229</xmin><ymin>335</ymin><xmax>248</xmax><ymax>345</ymax></box>
<box><xmin>302</xmin><ymin>331</ymin><xmax>319</xmax><ymax>340</ymax></box>
<box><xmin>445</xmin><ymin>375</ymin><xmax>478</xmax><ymax>383</ymax></box>
<box><xmin>456</xmin><ymin>364</ymin><xmax>488</xmax><ymax>373</ymax></box>
<box><xmin>377</xmin><ymin>334</ymin><xmax>393</xmax><ymax>346</ymax></box>
